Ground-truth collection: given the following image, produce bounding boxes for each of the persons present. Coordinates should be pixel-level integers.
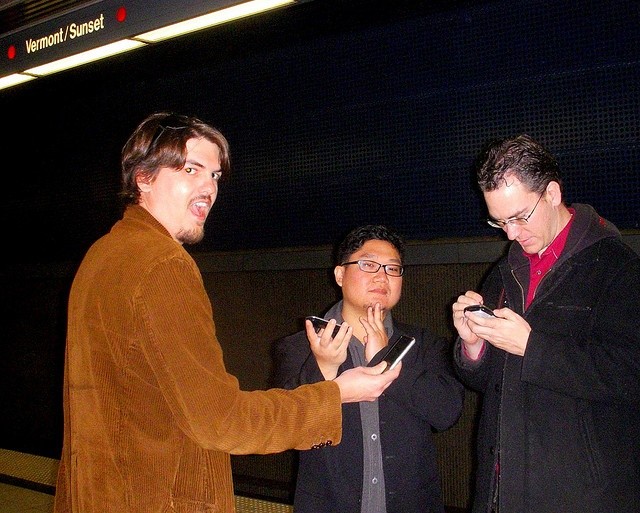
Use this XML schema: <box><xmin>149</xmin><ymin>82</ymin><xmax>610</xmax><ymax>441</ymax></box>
<box><xmin>270</xmin><ymin>225</ymin><xmax>464</xmax><ymax>512</ymax></box>
<box><xmin>450</xmin><ymin>134</ymin><xmax>640</xmax><ymax>513</ymax></box>
<box><xmin>52</xmin><ymin>113</ymin><xmax>402</xmax><ymax>513</ymax></box>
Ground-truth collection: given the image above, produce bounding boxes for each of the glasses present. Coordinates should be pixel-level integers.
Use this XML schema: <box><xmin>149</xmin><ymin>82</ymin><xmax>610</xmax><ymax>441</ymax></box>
<box><xmin>487</xmin><ymin>190</ymin><xmax>546</xmax><ymax>229</ymax></box>
<box><xmin>341</xmin><ymin>260</ymin><xmax>405</xmax><ymax>277</ymax></box>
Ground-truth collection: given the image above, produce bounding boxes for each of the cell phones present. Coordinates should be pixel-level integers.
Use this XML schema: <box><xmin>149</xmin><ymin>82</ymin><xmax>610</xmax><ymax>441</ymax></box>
<box><xmin>464</xmin><ymin>305</ymin><xmax>494</xmax><ymax>316</ymax></box>
<box><xmin>372</xmin><ymin>335</ymin><xmax>417</xmax><ymax>374</ymax></box>
<box><xmin>305</xmin><ymin>315</ymin><xmax>342</xmax><ymax>334</ymax></box>
